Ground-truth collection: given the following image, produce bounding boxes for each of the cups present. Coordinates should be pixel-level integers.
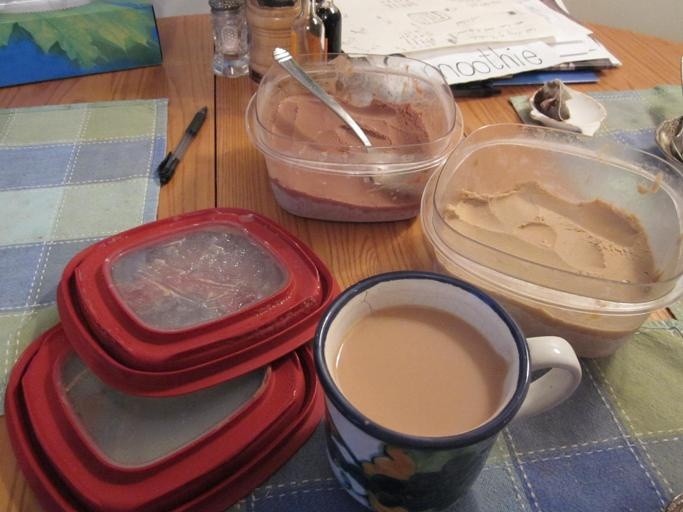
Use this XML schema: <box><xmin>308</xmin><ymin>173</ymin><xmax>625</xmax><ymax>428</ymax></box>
<box><xmin>314</xmin><ymin>271</ymin><xmax>582</xmax><ymax>512</ymax></box>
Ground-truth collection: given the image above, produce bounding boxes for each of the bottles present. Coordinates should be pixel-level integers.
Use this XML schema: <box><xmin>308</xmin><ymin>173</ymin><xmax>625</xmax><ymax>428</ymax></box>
<box><xmin>208</xmin><ymin>1</ymin><xmax>249</xmax><ymax>78</ymax></box>
<box><xmin>315</xmin><ymin>0</ymin><xmax>342</xmax><ymax>60</ymax></box>
<box><xmin>290</xmin><ymin>0</ymin><xmax>325</xmax><ymax>65</ymax></box>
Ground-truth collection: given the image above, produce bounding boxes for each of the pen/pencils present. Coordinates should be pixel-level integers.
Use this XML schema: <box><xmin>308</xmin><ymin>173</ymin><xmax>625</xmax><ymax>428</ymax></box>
<box><xmin>156</xmin><ymin>104</ymin><xmax>207</xmax><ymax>185</ymax></box>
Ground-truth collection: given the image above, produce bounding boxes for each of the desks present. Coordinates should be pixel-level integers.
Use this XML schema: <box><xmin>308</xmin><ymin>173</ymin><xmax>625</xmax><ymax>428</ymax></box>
<box><xmin>0</xmin><ymin>14</ymin><xmax>682</xmax><ymax>512</ymax></box>
